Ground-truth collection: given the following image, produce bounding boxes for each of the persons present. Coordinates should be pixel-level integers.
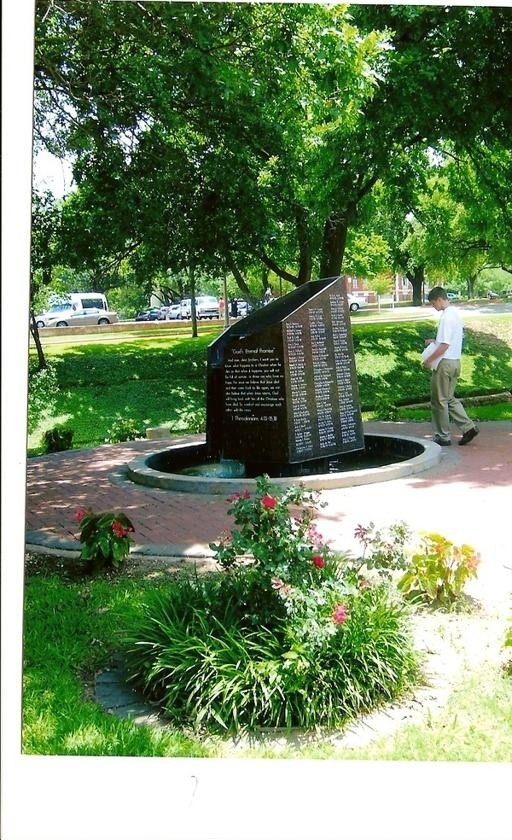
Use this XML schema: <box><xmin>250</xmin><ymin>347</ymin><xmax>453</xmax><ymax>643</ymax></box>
<box><xmin>422</xmin><ymin>287</ymin><xmax>480</xmax><ymax>446</ymax></box>
<box><xmin>219</xmin><ymin>297</ymin><xmax>225</xmax><ymax>319</ymax></box>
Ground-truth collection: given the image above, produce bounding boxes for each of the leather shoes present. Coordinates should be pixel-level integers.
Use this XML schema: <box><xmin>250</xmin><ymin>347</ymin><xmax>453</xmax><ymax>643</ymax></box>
<box><xmin>432</xmin><ymin>437</ymin><xmax>452</xmax><ymax>446</ymax></box>
<box><xmin>459</xmin><ymin>426</ymin><xmax>480</xmax><ymax>445</ymax></box>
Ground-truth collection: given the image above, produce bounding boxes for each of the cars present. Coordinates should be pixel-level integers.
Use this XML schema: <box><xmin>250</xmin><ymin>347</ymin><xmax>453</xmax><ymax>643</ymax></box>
<box><xmin>135</xmin><ymin>295</ymin><xmax>274</xmax><ymax>322</ymax></box>
<box><xmin>447</xmin><ymin>293</ymin><xmax>459</xmax><ymax>300</ymax></box>
<box><xmin>347</xmin><ymin>294</ymin><xmax>368</xmax><ymax>312</ymax></box>
<box><xmin>29</xmin><ymin>292</ymin><xmax>119</xmax><ymax>330</ymax></box>
